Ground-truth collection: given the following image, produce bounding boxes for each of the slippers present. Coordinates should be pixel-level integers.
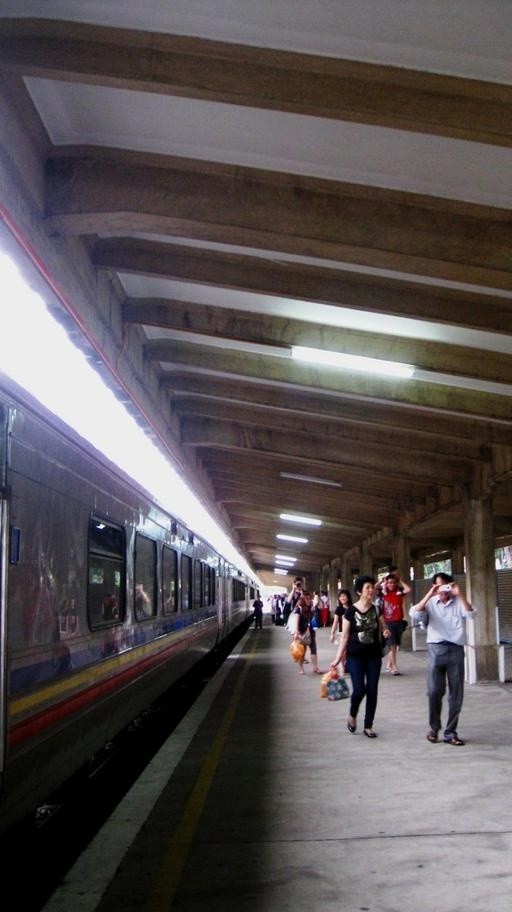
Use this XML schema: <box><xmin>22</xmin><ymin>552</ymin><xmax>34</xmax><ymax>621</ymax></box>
<box><xmin>391</xmin><ymin>669</ymin><xmax>401</xmax><ymax>675</ymax></box>
<box><xmin>385</xmin><ymin>666</ymin><xmax>393</xmax><ymax>673</ymax></box>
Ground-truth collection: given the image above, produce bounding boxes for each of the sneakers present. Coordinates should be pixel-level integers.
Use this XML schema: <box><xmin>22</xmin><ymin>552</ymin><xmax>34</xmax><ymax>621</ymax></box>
<box><xmin>347</xmin><ymin>721</ymin><xmax>357</xmax><ymax>733</ymax></box>
<box><xmin>364</xmin><ymin>727</ymin><xmax>378</xmax><ymax>738</ymax></box>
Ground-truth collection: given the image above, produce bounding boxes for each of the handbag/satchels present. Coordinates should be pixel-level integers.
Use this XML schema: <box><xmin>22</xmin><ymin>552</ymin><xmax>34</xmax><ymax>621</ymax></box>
<box><xmin>380</xmin><ymin>633</ymin><xmax>391</xmax><ymax>658</ymax></box>
<box><xmin>296</xmin><ymin>628</ymin><xmax>311</xmax><ymax>645</ymax></box>
<box><xmin>327</xmin><ymin>668</ymin><xmax>350</xmax><ymax>700</ymax></box>
<box><xmin>285</xmin><ymin>613</ymin><xmax>301</xmax><ymax>635</ymax></box>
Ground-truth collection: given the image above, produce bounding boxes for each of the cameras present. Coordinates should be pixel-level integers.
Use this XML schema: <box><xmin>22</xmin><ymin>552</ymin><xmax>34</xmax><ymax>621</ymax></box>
<box><xmin>296</xmin><ymin>583</ymin><xmax>302</xmax><ymax>588</ymax></box>
<box><xmin>439</xmin><ymin>584</ymin><xmax>452</xmax><ymax>592</ymax></box>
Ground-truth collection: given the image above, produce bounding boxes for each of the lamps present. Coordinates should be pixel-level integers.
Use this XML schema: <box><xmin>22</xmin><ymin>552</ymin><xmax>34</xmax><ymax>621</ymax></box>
<box><xmin>290</xmin><ymin>345</ymin><xmax>416</xmax><ymax>380</ymax></box>
<box><xmin>278</xmin><ymin>469</ymin><xmax>343</xmax><ymax>488</ymax></box>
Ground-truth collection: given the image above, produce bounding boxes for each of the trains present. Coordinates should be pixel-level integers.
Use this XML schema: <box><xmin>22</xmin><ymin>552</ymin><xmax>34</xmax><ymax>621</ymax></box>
<box><xmin>0</xmin><ymin>367</ymin><xmax>264</xmax><ymax>849</ymax></box>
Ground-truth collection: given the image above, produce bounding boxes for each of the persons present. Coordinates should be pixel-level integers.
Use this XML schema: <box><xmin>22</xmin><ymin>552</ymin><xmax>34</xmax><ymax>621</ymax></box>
<box><xmin>98</xmin><ymin>578</ymin><xmax>176</xmax><ymax>621</ymax></box>
<box><xmin>253</xmin><ymin>594</ymin><xmax>265</xmax><ymax>631</ymax></box>
<box><xmin>381</xmin><ymin>566</ymin><xmax>412</xmax><ymax>596</ymax></box>
<box><xmin>332</xmin><ymin>576</ymin><xmax>394</xmax><ymax>738</ymax></box>
<box><xmin>408</xmin><ymin>571</ymin><xmax>480</xmax><ymax>741</ymax></box>
<box><xmin>332</xmin><ymin>588</ymin><xmax>354</xmax><ymax>681</ymax></box>
<box><xmin>373</xmin><ymin>573</ymin><xmax>413</xmax><ymax>675</ymax></box>
<box><xmin>267</xmin><ymin>576</ymin><xmax>332</xmax><ymax>628</ymax></box>
<box><xmin>291</xmin><ymin>590</ymin><xmax>323</xmax><ymax>674</ymax></box>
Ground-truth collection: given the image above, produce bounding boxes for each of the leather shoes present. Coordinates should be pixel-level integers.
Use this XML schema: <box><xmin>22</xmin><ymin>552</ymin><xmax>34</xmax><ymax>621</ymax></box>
<box><xmin>426</xmin><ymin>726</ymin><xmax>439</xmax><ymax>741</ymax></box>
<box><xmin>444</xmin><ymin>735</ymin><xmax>464</xmax><ymax>745</ymax></box>
